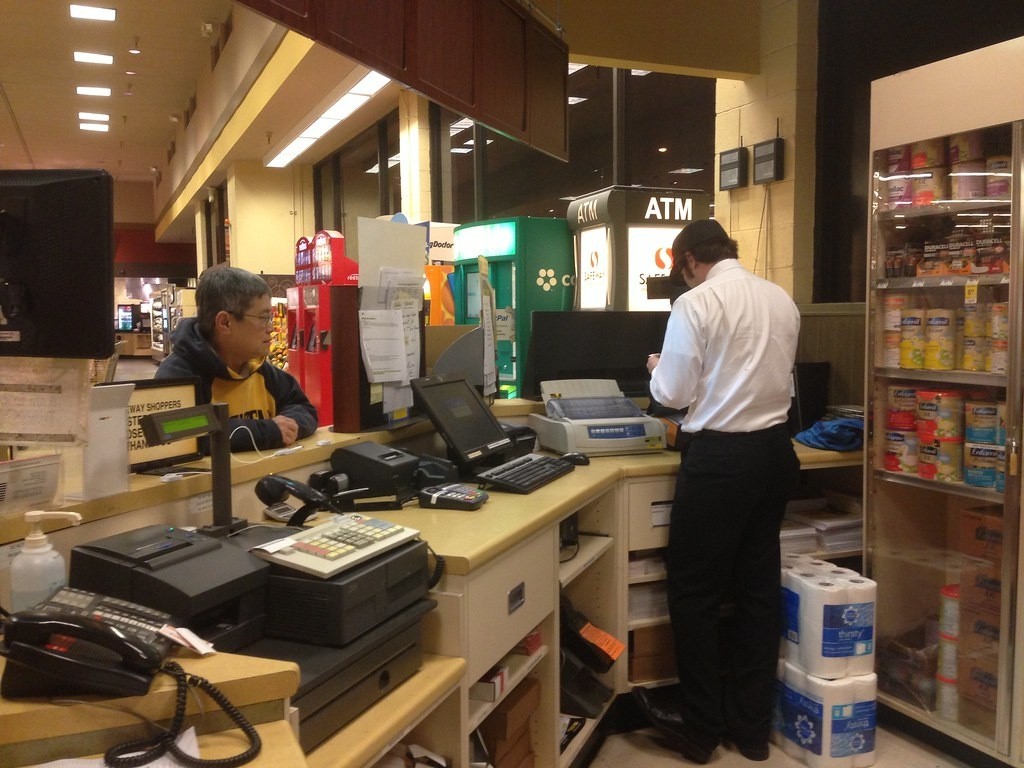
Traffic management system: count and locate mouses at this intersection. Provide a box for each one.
[559,452,590,465]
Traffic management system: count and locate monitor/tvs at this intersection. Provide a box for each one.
[409,371,514,483]
[0,168,114,360]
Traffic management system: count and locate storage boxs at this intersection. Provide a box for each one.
[11,378,137,502]
[480,676,542,740]
[628,658,679,683]
[508,627,542,656]
[490,718,530,766]
[630,623,676,658]
[495,729,531,768]
[517,751,535,768]
[470,662,511,703]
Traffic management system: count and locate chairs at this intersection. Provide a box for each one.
[91,339,128,383]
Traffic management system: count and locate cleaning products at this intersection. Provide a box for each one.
[7,509,85,616]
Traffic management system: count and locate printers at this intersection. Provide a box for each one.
[528,379,666,457]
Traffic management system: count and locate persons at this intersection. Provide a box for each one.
[630,220,801,765]
[154,265,318,456]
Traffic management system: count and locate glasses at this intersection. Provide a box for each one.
[225,309,272,326]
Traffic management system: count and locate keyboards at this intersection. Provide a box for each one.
[251,512,421,580]
[475,453,575,493]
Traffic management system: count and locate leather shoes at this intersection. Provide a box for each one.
[721,714,769,762]
[632,686,713,764]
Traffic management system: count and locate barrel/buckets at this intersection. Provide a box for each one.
[881,293,1008,372]
[878,384,1009,493]
[882,136,1011,201]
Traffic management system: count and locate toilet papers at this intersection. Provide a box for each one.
[769,551,880,768]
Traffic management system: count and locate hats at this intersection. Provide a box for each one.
[668,219,727,284]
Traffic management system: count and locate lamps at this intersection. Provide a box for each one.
[169,111,182,124]
[150,164,159,176]
[200,18,218,39]
[207,185,217,203]
[127,36,142,55]
[122,83,132,95]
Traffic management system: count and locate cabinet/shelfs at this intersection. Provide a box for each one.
[859,35,1024,768]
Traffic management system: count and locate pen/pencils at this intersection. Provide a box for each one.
[287,445,303,451]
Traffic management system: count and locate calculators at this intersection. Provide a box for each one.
[262,502,318,523]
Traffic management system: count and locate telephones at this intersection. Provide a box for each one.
[0,584,181,696]
